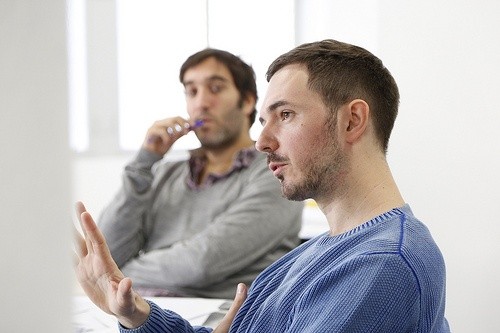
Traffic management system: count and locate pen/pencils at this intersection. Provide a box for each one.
[160,120,204,144]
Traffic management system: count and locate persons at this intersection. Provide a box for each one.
[71,39,451,333]
[96,49,305,300]
